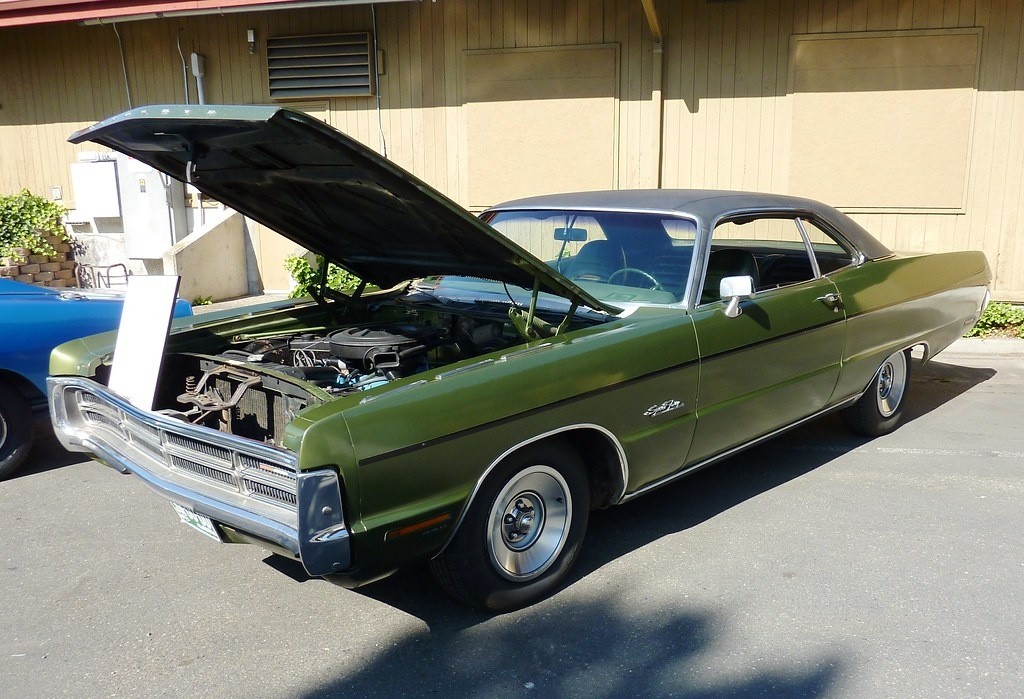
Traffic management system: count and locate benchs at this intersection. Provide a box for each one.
[756,254,835,287]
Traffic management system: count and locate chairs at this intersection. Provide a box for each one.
[563,240,627,286]
[678,248,759,305]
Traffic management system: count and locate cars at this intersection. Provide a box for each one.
[0,278,194,484]
[43,100,990,612]
